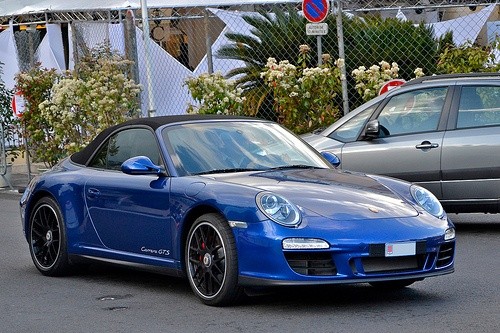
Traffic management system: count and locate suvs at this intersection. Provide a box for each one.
[298,71,499,213]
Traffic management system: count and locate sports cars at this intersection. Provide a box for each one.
[19,116,456,305]
